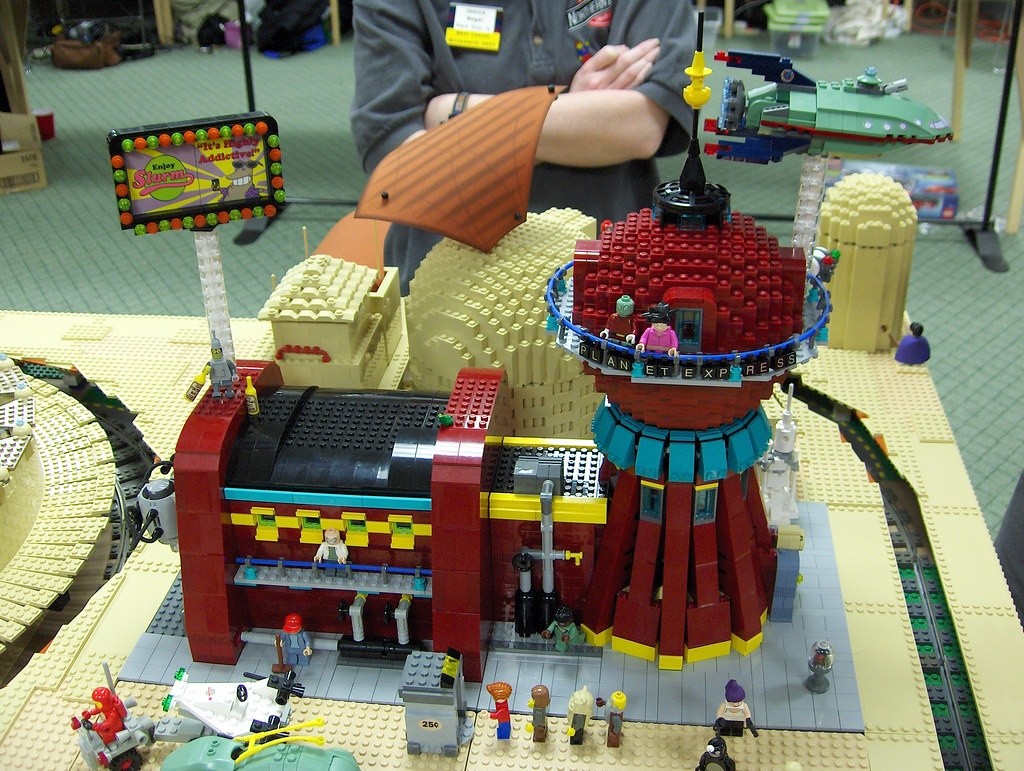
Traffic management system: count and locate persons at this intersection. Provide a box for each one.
[347,0,701,293]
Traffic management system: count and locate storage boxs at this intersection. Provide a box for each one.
[0,113,47,194]
[768,20,822,60]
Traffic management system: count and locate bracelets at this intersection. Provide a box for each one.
[448,90,470,117]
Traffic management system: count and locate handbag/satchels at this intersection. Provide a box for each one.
[198,14,230,46]
[51,32,120,68]
[257,0,329,57]
